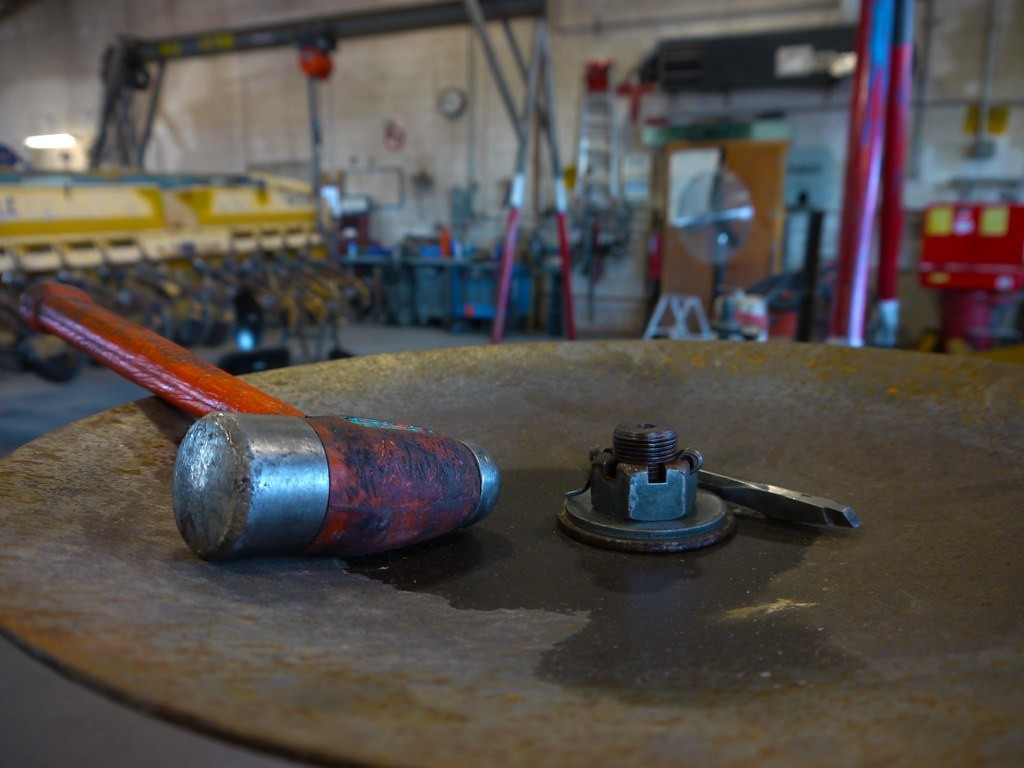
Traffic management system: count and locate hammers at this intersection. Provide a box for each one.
[14,274,502,565]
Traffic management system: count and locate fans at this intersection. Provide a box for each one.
[677,169,752,327]
